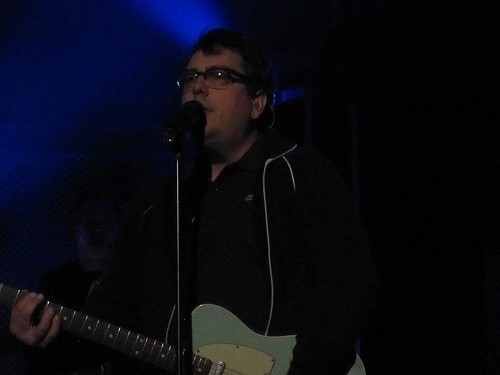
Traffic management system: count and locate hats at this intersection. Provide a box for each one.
[202,28,276,130]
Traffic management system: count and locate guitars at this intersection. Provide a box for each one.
[0,280,375,375]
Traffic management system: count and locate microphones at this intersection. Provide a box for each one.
[161,100,204,144]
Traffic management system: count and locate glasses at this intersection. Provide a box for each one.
[176,67,253,89]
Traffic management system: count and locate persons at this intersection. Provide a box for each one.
[4,27,378,375]
